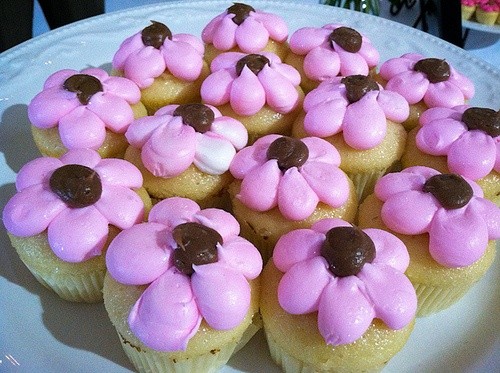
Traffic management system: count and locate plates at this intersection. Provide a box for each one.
[0,0,500,373]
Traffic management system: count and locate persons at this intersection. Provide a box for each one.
[0,0,107,52]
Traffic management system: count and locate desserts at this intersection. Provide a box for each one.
[0,1,499,373]
